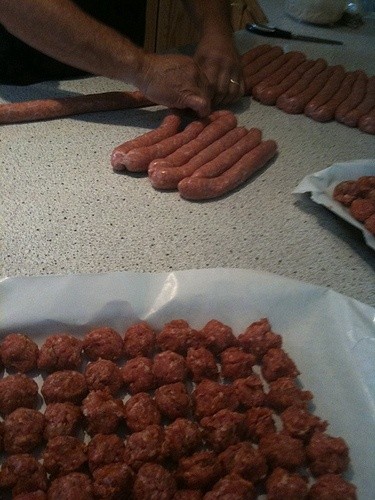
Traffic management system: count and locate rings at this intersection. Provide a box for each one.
[229,78,241,85]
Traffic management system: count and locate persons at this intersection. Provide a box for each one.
[1,0,248,118]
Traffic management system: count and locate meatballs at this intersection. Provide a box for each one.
[0,317,358,500]
[333,176,375,239]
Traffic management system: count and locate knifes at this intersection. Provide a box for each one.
[246,23,344,46]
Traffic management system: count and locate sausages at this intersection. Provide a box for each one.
[0,42,374,199]
